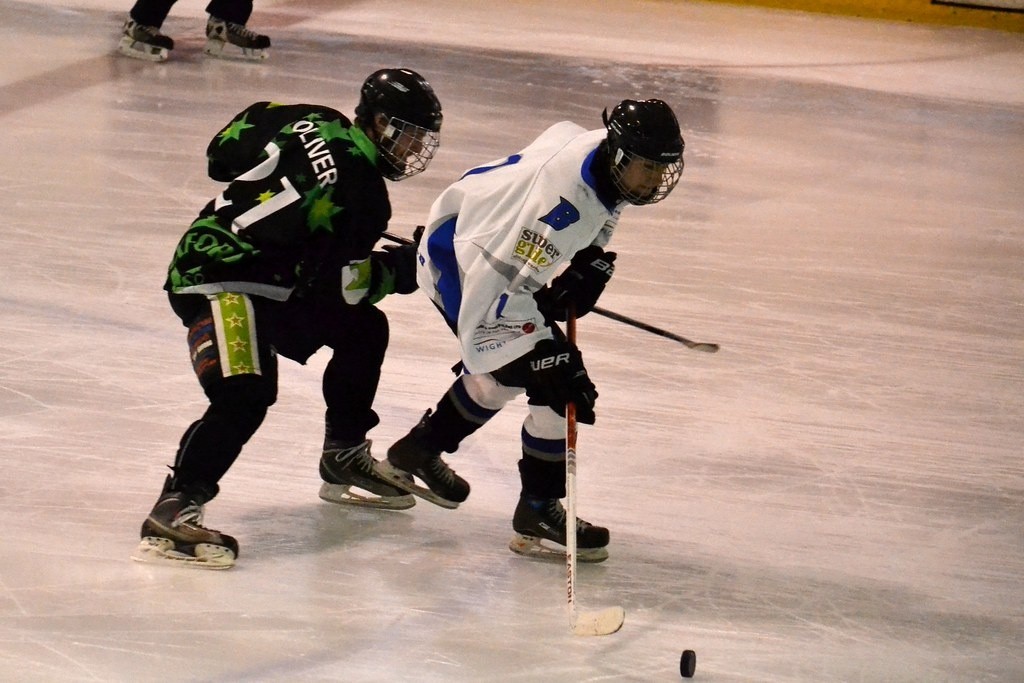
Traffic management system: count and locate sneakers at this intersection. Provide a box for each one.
[115,17,176,62]
[318,410,417,510]
[204,14,271,60]
[372,414,471,510]
[129,473,240,571]
[509,496,610,564]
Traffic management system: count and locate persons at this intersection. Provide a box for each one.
[118,0,271,60]
[373,99,686,561]
[140,69,443,569]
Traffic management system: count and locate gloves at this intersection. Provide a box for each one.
[537,244,616,322]
[392,225,425,295]
[549,366,599,425]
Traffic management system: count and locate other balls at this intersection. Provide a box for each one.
[680,650,698,678]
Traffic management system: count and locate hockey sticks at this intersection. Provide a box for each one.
[382,229,723,354]
[566,304,626,637]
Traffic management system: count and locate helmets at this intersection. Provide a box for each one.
[353,67,444,183]
[606,99,686,207]
